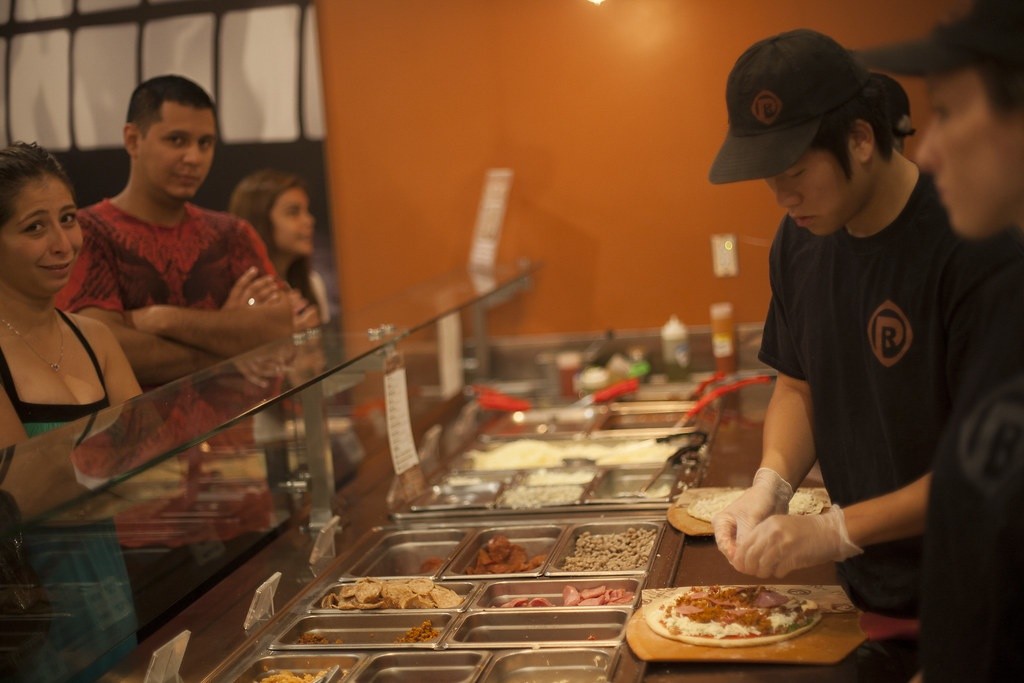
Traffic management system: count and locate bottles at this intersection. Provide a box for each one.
[661,314,689,378]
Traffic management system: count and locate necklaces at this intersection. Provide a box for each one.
[0,301,65,372]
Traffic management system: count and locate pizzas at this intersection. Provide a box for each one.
[690,491,823,522]
[646,588,822,646]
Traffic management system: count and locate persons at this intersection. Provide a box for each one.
[53,73,294,683]
[225,169,332,559]
[711,28,959,682]
[850,0,1024,682]
[0,137,142,683]
[860,72,919,159]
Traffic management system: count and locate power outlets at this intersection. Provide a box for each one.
[708,234,738,277]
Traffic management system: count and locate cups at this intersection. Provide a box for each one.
[710,303,735,373]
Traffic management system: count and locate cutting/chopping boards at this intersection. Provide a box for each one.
[667,488,830,535]
[624,601,922,666]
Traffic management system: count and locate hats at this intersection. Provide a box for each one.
[710,29,869,184]
[859,0,1024,108]
[868,72,917,136]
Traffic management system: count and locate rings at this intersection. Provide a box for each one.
[247,297,257,307]
